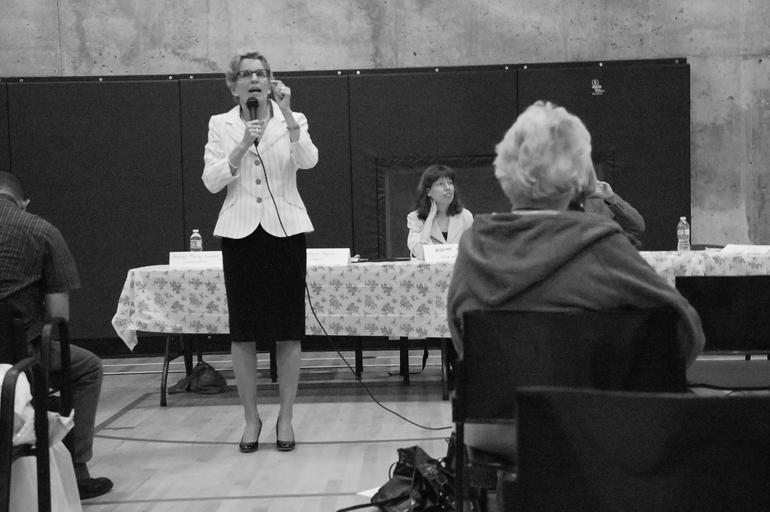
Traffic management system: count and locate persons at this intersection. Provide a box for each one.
[407,162,474,260]
[450,97,701,480]
[205,50,319,453]
[573,172,646,248]
[0,166,114,500]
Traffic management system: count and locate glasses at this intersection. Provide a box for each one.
[229,66,272,81]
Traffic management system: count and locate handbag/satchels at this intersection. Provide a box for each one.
[369,442,475,512]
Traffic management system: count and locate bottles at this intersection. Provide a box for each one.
[189,229,202,251]
[676,216,690,250]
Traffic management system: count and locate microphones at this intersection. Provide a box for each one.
[246,96,261,147]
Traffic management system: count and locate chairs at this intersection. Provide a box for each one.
[167,249,225,385]
[497,387,770,512]
[0,357,51,512]
[269,246,365,382]
[450,310,683,511]
[0,304,72,417]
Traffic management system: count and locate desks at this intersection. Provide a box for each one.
[110,249,769,406]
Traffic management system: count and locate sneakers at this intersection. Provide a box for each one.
[77,477,114,500]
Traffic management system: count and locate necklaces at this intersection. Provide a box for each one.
[240,109,271,124]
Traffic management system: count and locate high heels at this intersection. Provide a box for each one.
[273,415,298,451]
[238,419,263,454]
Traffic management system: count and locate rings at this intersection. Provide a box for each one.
[256,129,259,134]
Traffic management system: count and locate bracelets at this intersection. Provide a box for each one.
[286,126,300,132]
[228,158,240,170]
